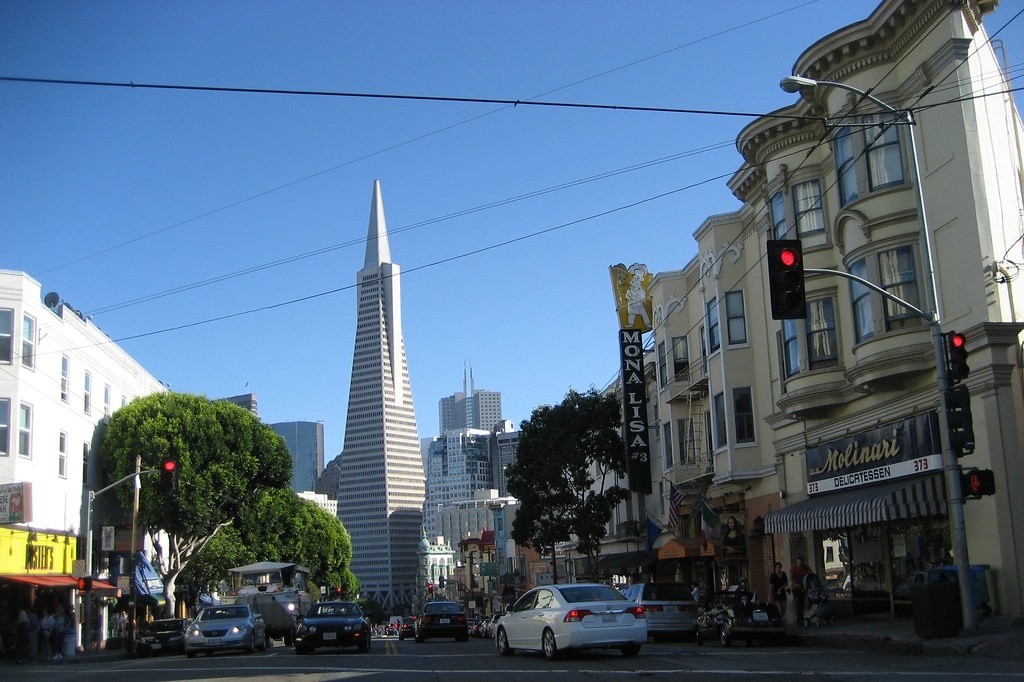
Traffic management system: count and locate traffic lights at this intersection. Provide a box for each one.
[966,468,996,496]
[945,382,975,457]
[948,331,970,379]
[766,238,809,320]
[160,456,179,496]
[428,583,433,593]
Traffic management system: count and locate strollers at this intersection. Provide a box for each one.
[801,573,835,627]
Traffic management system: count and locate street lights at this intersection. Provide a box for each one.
[778,74,976,636]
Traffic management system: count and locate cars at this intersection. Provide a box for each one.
[398,616,417,640]
[467,614,506,639]
[295,600,371,654]
[414,601,468,643]
[494,582,648,661]
[386,622,397,636]
[695,589,785,649]
[184,603,265,658]
[136,617,193,659]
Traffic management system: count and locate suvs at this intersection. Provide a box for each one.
[619,582,697,642]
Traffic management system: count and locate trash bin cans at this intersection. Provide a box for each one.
[911,570,962,639]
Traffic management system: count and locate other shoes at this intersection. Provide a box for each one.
[52,653,63,660]
[40,655,52,662]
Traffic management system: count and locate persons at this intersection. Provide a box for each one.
[41,606,72,662]
[15,610,32,664]
[118,611,128,636]
[690,582,700,602]
[768,562,790,618]
[789,556,813,627]
[806,580,827,601]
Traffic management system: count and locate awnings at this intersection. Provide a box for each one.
[130,586,167,608]
[658,538,723,560]
[764,469,948,534]
[5,574,121,597]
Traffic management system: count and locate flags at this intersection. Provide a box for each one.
[645,515,663,556]
[700,502,718,553]
[668,482,687,528]
